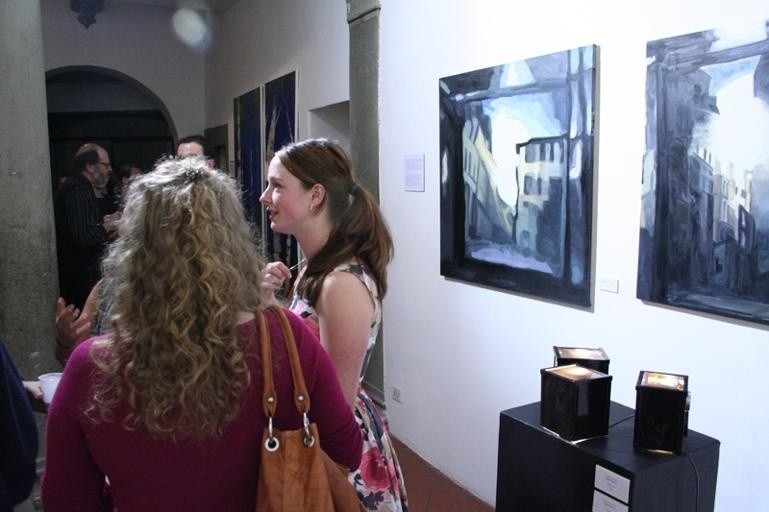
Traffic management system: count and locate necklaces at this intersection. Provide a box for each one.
[287,257,312,271]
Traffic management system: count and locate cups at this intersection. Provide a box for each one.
[38,371,64,414]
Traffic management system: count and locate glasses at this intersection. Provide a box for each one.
[95,162,110,168]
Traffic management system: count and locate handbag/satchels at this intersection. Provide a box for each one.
[255,304,369,512]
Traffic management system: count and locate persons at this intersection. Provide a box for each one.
[0,339,58,512]
[255,138,412,512]
[38,155,366,512]
